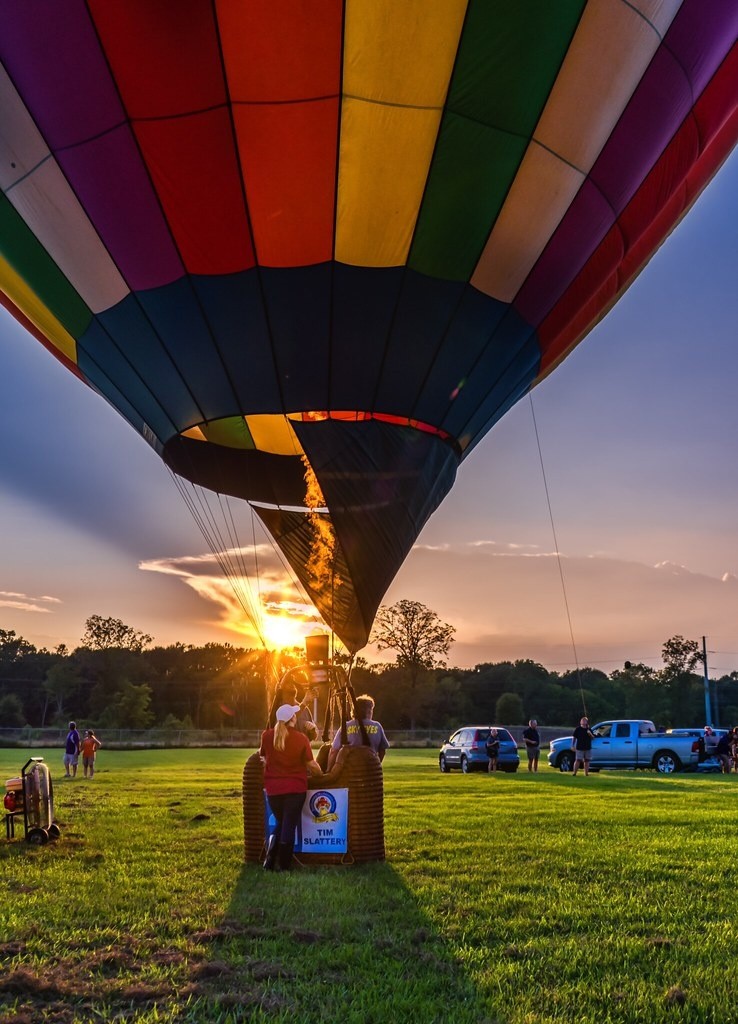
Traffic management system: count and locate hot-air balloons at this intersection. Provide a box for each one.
[4,1,736,862]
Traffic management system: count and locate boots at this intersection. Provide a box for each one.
[276,848,304,872]
[263,835,278,868]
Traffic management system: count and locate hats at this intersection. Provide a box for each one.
[276,703,300,724]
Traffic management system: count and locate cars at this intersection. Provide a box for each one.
[438,727,519,774]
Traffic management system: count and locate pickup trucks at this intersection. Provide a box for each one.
[548,720,704,773]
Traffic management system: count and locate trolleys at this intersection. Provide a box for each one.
[3,756,60,845]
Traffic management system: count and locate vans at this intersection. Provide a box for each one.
[667,728,729,754]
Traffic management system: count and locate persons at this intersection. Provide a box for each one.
[571,717,594,777]
[269,674,319,744]
[63,722,80,778]
[324,694,389,774]
[78,729,101,781]
[703,724,738,773]
[486,729,500,773]
[522,720,540,773]
[257,705,322,872]
